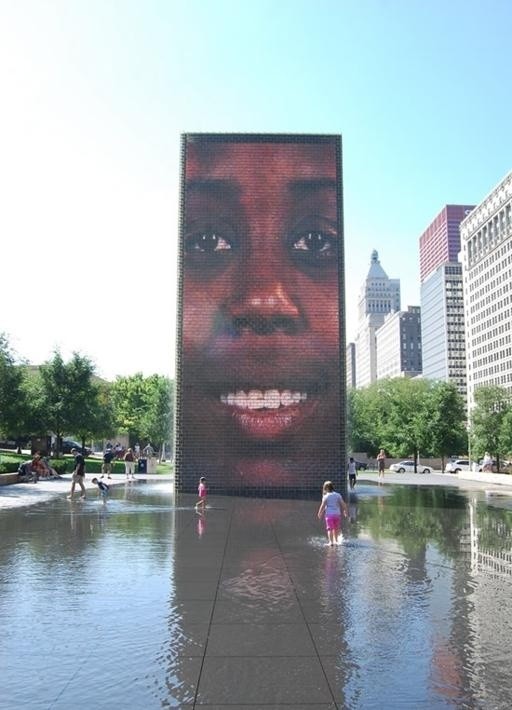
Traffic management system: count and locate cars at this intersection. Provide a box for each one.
[348,458,369,471]
[51,441,92,457]
[390,461,433,474]
[446,460,512,473]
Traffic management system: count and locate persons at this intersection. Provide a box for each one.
[122,447,137,480]
[105,440,155,460]
[100,449,113,479]
[193,476,208,510]
[376,449,387,479]
[91,477,109,505]
[194,511,209,541]
[17,448,63,482]
[346,457,358,490]
[66,447,87,501]
[317,481,348,547]
[177,136,342,502]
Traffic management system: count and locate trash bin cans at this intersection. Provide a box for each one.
[138,459,147,474]
[147,457,157,473]
